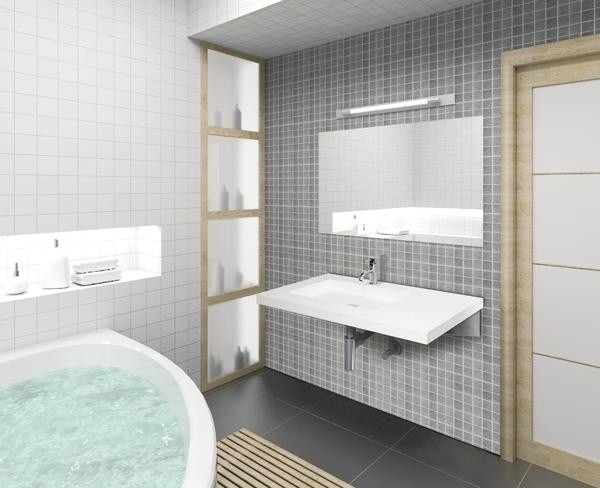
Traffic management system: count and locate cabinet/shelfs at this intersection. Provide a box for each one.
[199,40,268,392]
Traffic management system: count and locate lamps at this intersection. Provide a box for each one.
[335,93,456,121]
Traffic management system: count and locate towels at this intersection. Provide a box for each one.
[71,257,121,286]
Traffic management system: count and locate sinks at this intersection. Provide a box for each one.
[255,272,484,345]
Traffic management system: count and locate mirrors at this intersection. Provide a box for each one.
[317,114,485,249]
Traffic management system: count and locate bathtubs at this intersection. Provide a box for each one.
[0,327,218,488]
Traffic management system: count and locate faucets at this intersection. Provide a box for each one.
[359,257,377,284]
